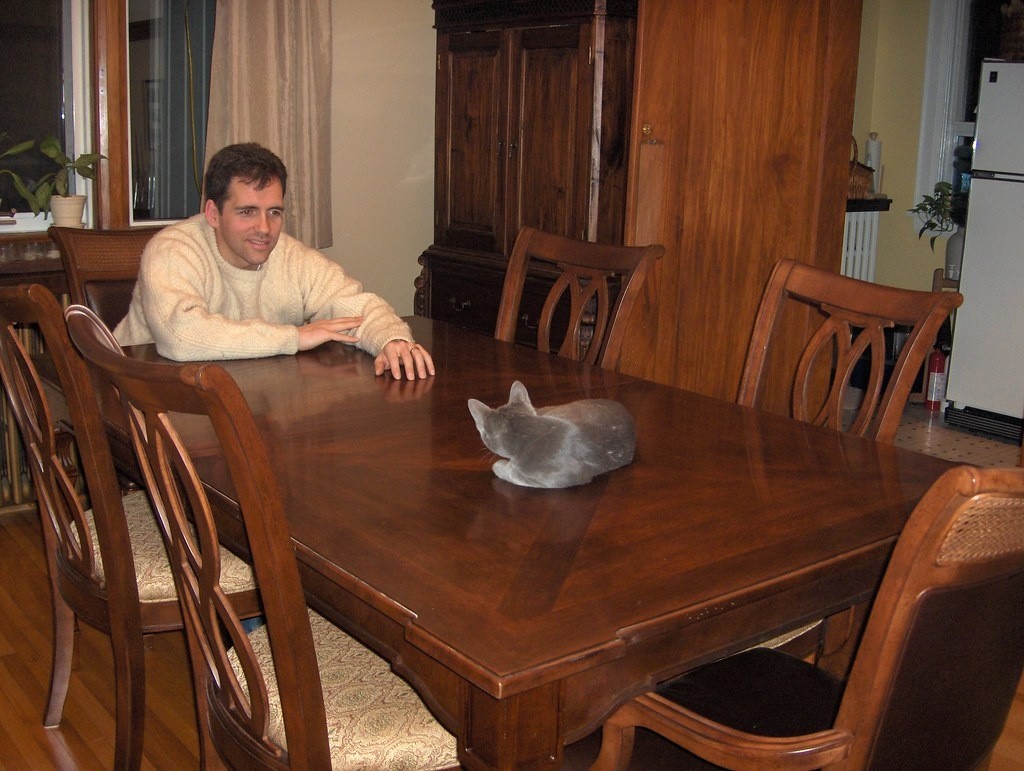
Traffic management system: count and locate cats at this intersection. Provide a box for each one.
[467,380,635,487]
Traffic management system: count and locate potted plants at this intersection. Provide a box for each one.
[909,180,966,280]
[0,134,109,228]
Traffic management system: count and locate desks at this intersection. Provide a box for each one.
[31,316,968,771]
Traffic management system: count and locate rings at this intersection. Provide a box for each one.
[410,348,420,353]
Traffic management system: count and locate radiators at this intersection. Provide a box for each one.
[840,211,880,283]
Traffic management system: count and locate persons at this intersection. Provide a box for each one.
[110,143,436,635]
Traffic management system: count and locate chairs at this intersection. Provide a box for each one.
[602,466,1024,771]
[0,281,263,771]
[738,260,964,646]
[59,303,460,771]
[492,227,666,367]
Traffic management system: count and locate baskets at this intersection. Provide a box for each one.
[846,135,874,199]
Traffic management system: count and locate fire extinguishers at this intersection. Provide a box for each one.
[924,344,946,411]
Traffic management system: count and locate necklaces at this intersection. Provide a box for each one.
[256,264,261,270]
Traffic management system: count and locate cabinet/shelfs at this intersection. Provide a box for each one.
[0,229,106,516]
[425,0,863,423]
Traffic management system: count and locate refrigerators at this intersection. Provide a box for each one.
[945,57,1024,443]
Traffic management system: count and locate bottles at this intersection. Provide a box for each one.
[864,132,882,195]
